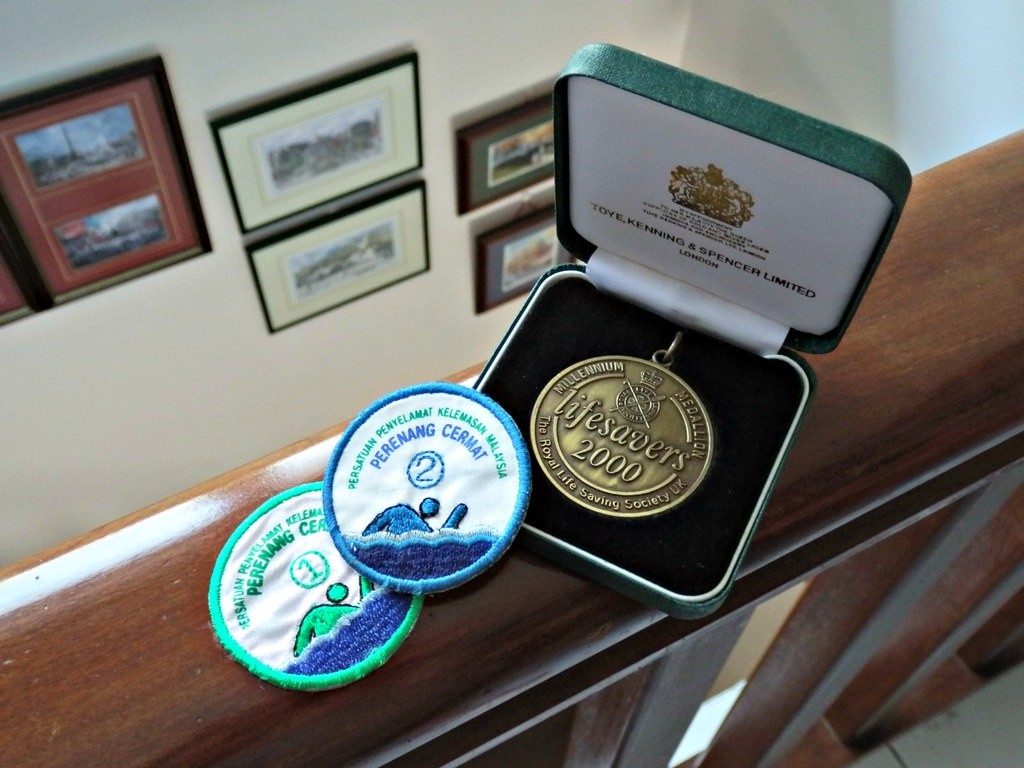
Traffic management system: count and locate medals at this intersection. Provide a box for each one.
[529,331,713,517]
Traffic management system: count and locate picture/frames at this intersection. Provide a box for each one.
[245,179,430,333]
[456,92,555,216]
[0,54,214,328]
[209,52,424,235]
[474,201,577,315]
